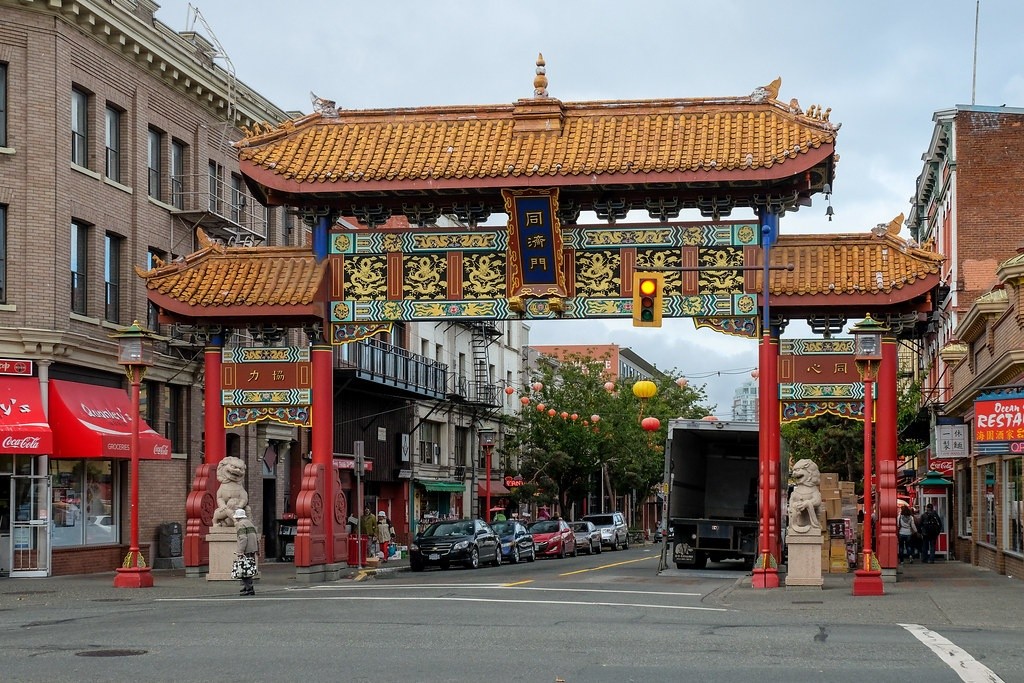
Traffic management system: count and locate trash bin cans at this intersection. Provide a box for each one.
[347,534,368,567]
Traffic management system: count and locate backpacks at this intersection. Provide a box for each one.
[923,512,938,536]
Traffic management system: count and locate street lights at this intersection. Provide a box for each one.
[475,421,498,522]
[846,313,892,597]
[107,320,172,588]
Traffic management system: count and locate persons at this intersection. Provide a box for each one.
[231,509,258,596]
[539,508,550,520]
[551,512,562,521]
[896,504,943,564]
[356,506,395,563]
[857,510,864,523]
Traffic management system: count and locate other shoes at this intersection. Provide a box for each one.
[910,555,913,564]
[930,561,934,564]
[239,586,246,592]
[240,589,256,597]
[901,562,904,565]
[382,560,387,563]
[921,560,928,563]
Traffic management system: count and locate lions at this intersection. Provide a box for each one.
[789,459,820,524]
[214,456,248,525]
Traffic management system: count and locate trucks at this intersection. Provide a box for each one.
[656,418,790,570]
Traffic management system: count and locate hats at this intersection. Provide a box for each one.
[233,509,247,518]
[539,505,549,508]
[378,511,386,517]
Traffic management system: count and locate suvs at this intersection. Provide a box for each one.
[578,511,630,551]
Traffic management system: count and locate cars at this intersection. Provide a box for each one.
[566,521,602,555]
[525,519,578,559]
[486,520,536,564]
[408,518,503,572]
[654,521,674,542]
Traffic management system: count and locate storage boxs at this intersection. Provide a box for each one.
[817,474,864,573]
[389,546,407,561]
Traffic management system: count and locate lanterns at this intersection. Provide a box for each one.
[605,382,614,392]
[537,404,545,412]
[675,378,687,387]
[752,370,760,378]
[533,382,544,391]
[521,397,529,404]
[548,408,556,417]
[641,417,661,433]
[505,387,514,394]
[632,381,657,403]
[561,412,600,432]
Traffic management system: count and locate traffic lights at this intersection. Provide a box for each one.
[633,272,663,327]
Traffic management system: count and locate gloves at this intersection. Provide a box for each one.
[391,533,395,537]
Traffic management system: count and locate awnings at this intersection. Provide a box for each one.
[478,479,511,497]
[0,376,172,461]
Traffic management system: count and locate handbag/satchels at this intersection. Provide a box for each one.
[897,514,901,535]
[231,554,258,579]
[387,538,396,557]
